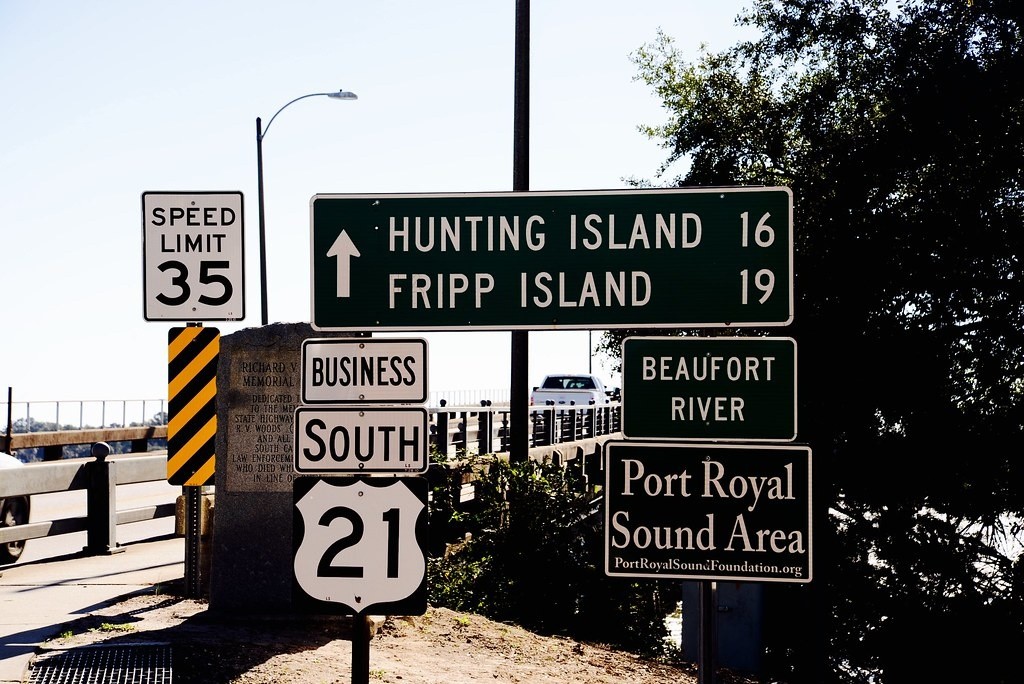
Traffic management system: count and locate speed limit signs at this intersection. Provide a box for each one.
[139,188,247,331]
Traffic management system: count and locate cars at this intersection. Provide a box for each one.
[530,374,615,416]
[0,436,34,563]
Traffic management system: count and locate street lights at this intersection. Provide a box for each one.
[253,88,359,328]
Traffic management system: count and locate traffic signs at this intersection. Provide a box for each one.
[307,183,802,330]
